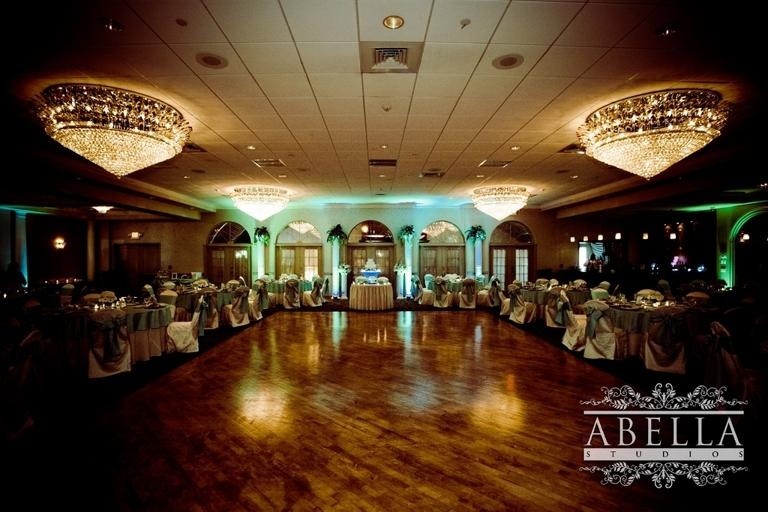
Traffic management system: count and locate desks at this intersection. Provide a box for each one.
[349,281,395,310]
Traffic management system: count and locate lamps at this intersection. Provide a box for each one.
[31,83,195,180]
[574,89,730,182]
[214,182,300,223]
[461,182,535,224]
